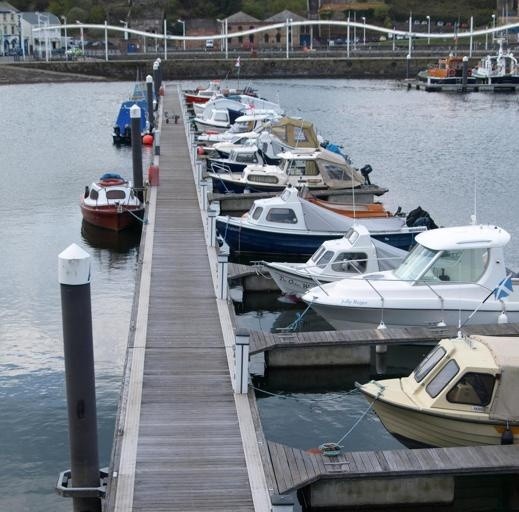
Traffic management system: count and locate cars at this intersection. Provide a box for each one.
[81,40,113,48]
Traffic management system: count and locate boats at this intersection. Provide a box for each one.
[79,173,144,232]
[415,37,519,91]
[113,83,157,143]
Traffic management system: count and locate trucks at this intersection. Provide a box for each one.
[62,37,80,48]
[206,40,213,48]
